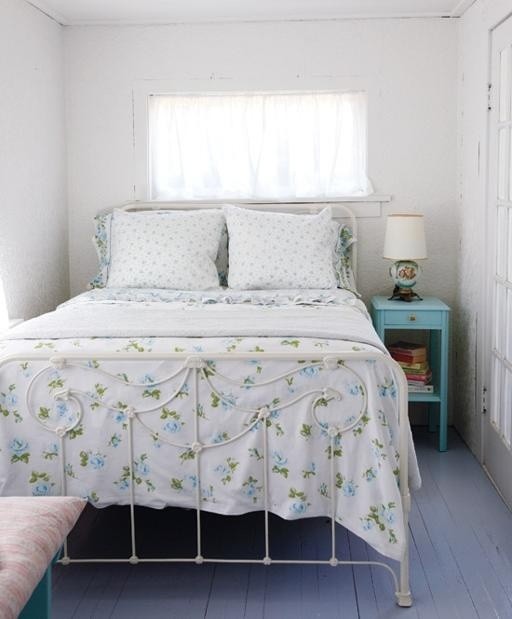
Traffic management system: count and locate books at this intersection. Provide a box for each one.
[387,341,434,393]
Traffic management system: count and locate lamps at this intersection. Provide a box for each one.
[381,212,426,301]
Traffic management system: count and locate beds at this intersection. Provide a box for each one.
[1,200,423,608]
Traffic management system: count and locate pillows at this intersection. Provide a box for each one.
[223,202,339,291]
[107,209,226,290]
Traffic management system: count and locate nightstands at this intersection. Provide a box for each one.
[373,295,452,453]
